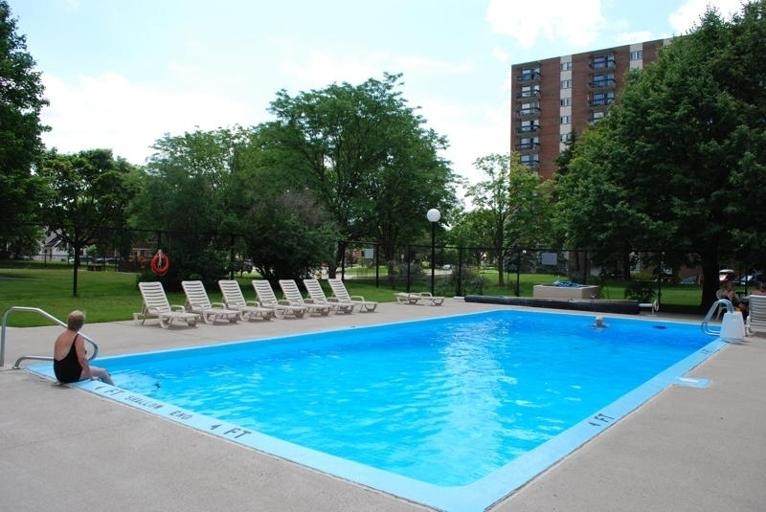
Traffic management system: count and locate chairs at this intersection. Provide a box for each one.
[394,291,444,306]
[715,289,741,320]
[133,278,379,329]
[746,295,766,337]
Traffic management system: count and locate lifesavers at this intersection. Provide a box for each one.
[151,255,169,277]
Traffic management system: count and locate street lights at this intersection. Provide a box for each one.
[426,208,441,296]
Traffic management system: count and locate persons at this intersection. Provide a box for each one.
[720,281,766,324]
[591,317,607,328]
[52,309,116,386]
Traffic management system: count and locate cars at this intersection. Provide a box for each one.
[719,269,766,290]
